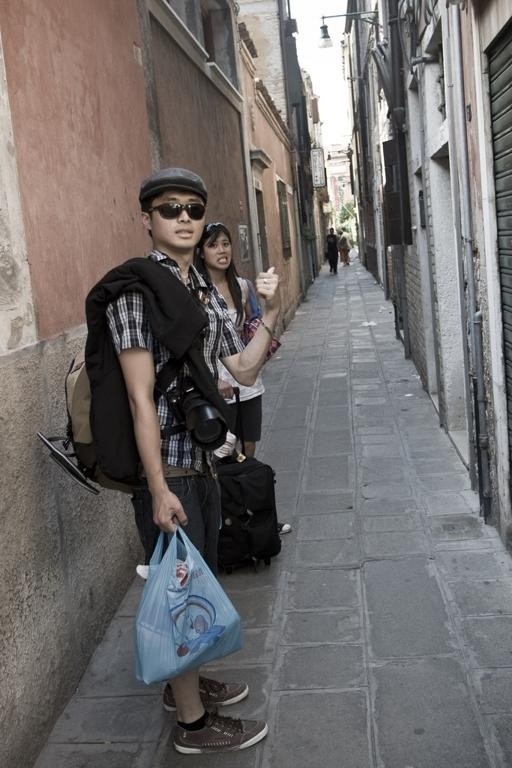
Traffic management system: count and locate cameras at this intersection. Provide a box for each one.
[167,376,227,451]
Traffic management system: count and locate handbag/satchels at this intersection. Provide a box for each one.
[242,317,283,362]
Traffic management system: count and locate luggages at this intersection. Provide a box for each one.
[213,385,282,573]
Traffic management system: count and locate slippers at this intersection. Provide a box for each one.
[37,433,102,498]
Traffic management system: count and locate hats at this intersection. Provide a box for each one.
[138,166,209,204]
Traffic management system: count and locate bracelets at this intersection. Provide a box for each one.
[259,318,273,340]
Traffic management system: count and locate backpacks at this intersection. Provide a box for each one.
[62,347,185,498]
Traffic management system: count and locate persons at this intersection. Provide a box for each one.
[193,220,293,535]
[325,226,340,275]
[84,165,278,757]
[336,231,350,267]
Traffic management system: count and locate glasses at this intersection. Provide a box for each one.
[145,200,206,221]
[205,222,225,234]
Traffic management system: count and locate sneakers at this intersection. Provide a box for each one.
[277,522,292,534]
[166,714,268,757]
[161,672,252,715]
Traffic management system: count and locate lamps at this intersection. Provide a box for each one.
[318,10,380,52]
